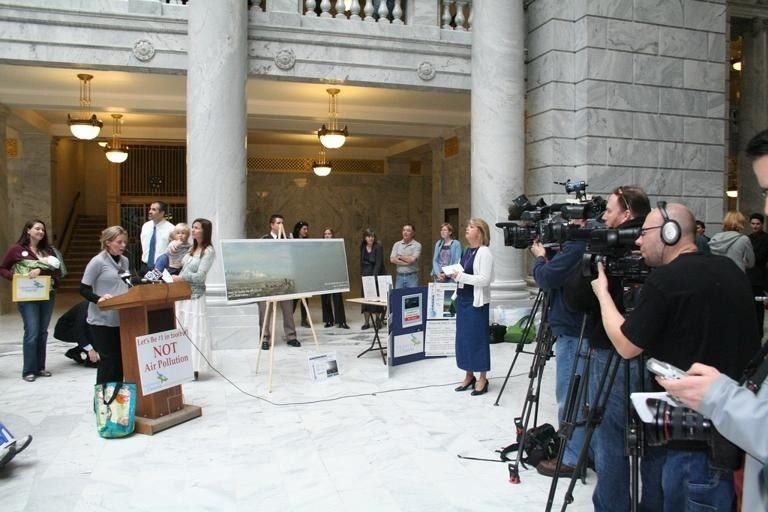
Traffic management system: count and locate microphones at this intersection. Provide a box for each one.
[132,277,161,283]
[118,269,135,287]
[140,269,157,280]
[150,266,167,283]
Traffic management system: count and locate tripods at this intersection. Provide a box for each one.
[494,287,544,406]
[560,300,644,512]
[509,299,556,484]
[545,311,587,512]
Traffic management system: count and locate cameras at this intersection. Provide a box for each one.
[644,397,743,471]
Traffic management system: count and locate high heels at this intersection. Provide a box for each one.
[470,378,489,396]
[455,376,476,391]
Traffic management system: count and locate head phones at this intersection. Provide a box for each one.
[657,201,681,246]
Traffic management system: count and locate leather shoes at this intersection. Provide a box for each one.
[300,319,312,329]
[338,321,351,330]
[23,374,35,382]
[323,320,335,328]
[37,370,51,376]
[287,339,301,348]
[261,341,270,351]
[361,321,381,330]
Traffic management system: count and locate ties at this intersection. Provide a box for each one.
[147,225,156,269]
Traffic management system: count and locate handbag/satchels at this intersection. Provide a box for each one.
[94,383,137,439]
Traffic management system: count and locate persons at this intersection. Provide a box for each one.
[154,222,191,276]
[0,218,60,382]
[79,226,131,412]
[531,127,768,512]
[0,421,32,470]
[449,217,493,396]
[53,301,100,368]
[175,217,214,380]
[138,201,176,277]
[260,214,461,351]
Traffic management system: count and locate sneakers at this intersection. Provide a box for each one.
[0,446,15,467]
[534,456,583,481]
[64,349,86,364]
[15,433,32,452]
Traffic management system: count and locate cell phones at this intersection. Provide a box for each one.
[647,358,694,381]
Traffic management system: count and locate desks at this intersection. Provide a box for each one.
[346,297,388,365]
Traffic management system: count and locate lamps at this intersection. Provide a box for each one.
[67,74,103,141]
[312,146,333,177]
[318,88,348,150]
[731,57,742,72]
[105,114,129,165]
[726,185,738,198]
